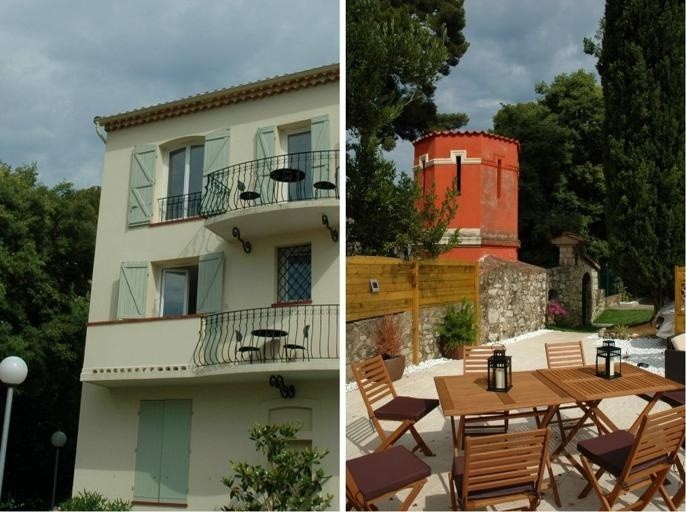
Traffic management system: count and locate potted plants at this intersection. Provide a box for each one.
[433,298,483,360]
[366,309,405,381]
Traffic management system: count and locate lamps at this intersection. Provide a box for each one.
[232,227,251,253]
[269,375,295,399]
[322,213,338,242]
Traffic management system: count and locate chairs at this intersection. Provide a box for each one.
[235,180,262,209]
[314,167,339,198]
[235,325,310,363]
[346,341,684,511]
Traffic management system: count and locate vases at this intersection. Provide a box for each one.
[546,311,557,326]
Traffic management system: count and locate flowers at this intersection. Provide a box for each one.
[548,299,567,317]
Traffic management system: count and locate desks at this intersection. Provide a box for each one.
[270,168,305,203]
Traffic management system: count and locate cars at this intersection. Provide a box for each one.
[653,297,684,339]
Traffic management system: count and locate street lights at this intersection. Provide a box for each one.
[0,356,28,497]
[51,431,67,508]
